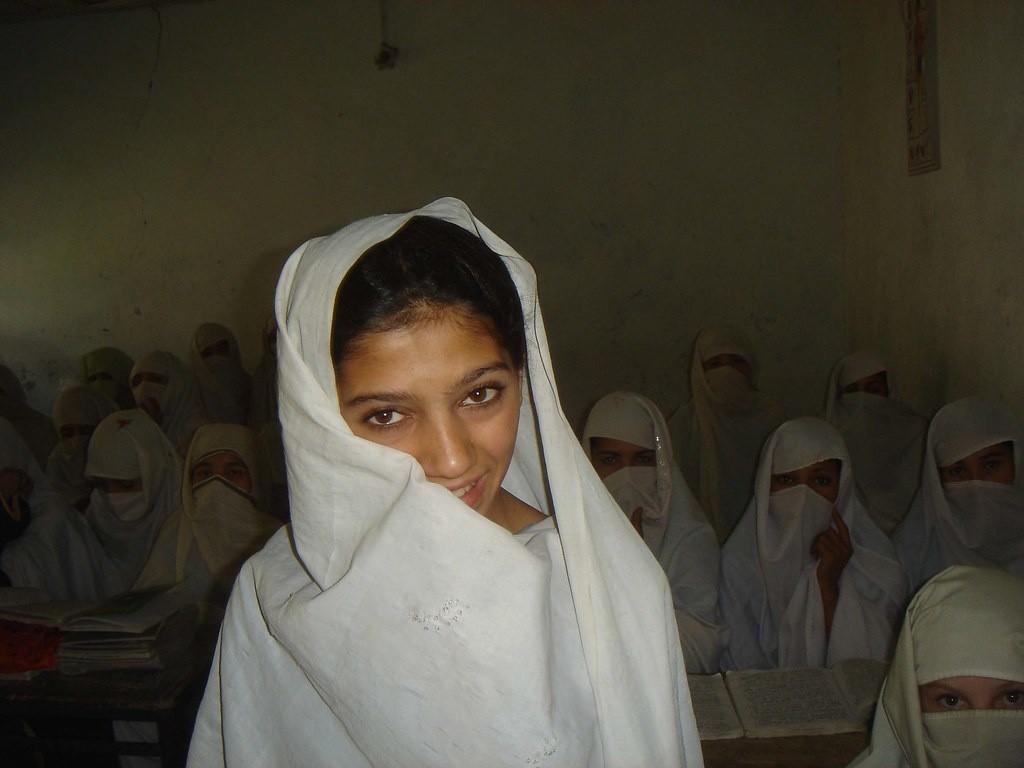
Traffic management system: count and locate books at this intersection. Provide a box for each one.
[0,587,197,711]
[690,656,893,741]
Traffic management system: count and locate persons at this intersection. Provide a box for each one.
[181,198,700,768]
[81,347,136,411]
[127,351,206,460]
[585,390,730,675]
[815,351,929,537]
[887,398,1024,599]
[83,409,181,768]
[0,365,58,474]
[132,423,286,631]
[666,324,788,546]
[0,417,108,601]
[717,416,909,674]
[180,324,252,425]
[848,565,1024,768]
[45,388,100,515]
[248,310,286,498]
